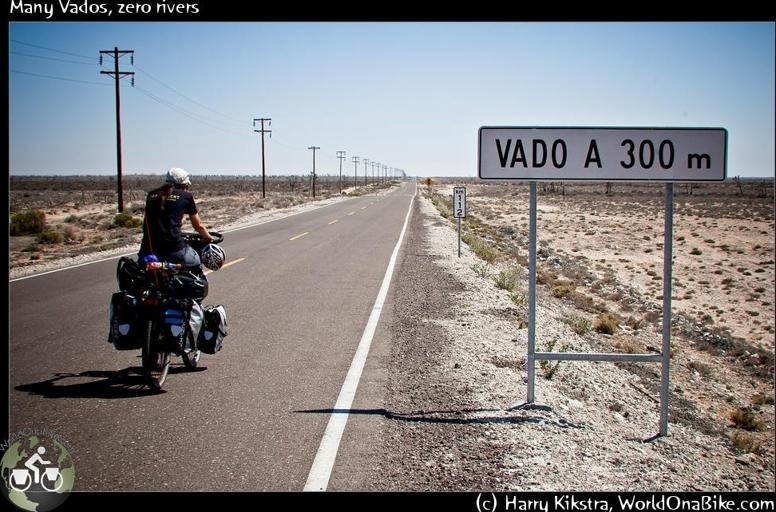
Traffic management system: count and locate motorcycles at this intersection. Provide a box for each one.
[106,230,229,396]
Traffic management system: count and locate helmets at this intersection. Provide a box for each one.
[201,243,226,271]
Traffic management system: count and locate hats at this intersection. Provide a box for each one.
[166,167,192,187]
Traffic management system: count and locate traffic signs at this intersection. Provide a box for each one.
[479,128,727,183]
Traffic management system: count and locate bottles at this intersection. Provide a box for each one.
[146,263,182,271]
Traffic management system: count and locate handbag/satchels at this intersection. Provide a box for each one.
[106,255,227,354]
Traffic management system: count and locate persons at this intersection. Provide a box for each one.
[138,168,214,268]
